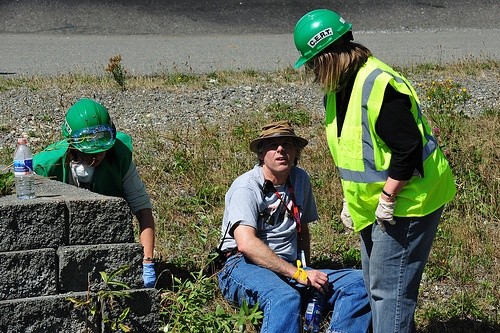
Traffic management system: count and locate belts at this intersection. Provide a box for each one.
[224,249,240,261]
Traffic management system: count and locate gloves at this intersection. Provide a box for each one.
[375,197,396,233]
[142,263,156,288]
[340,197,354,231]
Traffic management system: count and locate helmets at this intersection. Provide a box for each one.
[293,8,353,69]
[61,97,117,154]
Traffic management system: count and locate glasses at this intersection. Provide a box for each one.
[72,124,114,148]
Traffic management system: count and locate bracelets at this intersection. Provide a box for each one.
[292,260,306,281]
[142,257,156,261]
[382,189,397,198]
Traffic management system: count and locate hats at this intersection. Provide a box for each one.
[250,120,308,154]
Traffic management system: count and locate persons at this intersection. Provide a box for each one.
[293,9,458,333]
[32,98,157,288]
[216,119,373,333]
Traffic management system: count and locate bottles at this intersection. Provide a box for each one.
[304,292,322,333]
[14,138,35,200]
